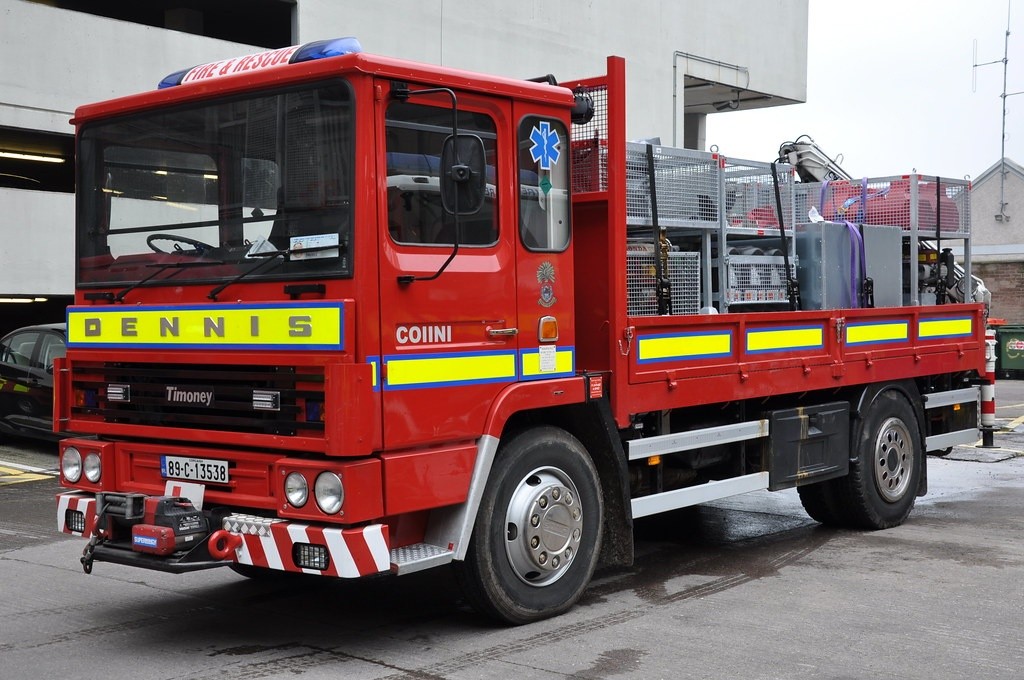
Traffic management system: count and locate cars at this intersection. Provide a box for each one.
[0,321,92,443]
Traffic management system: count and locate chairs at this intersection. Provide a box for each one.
[48,343,66,366]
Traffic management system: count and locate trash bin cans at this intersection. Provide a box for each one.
[985,317,1024,380]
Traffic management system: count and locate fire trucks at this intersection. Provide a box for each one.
[50,35,1000,622]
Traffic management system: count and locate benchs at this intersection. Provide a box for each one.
[2,341,36,366]
